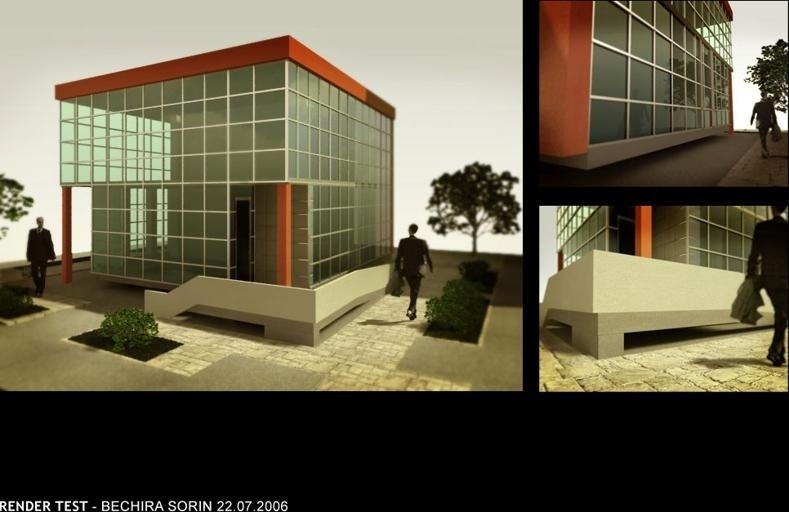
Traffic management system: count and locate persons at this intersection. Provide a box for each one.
[24,216,58,300]
[744,204,788,367]
[392,223,435,320]
[749,89,780,159]
